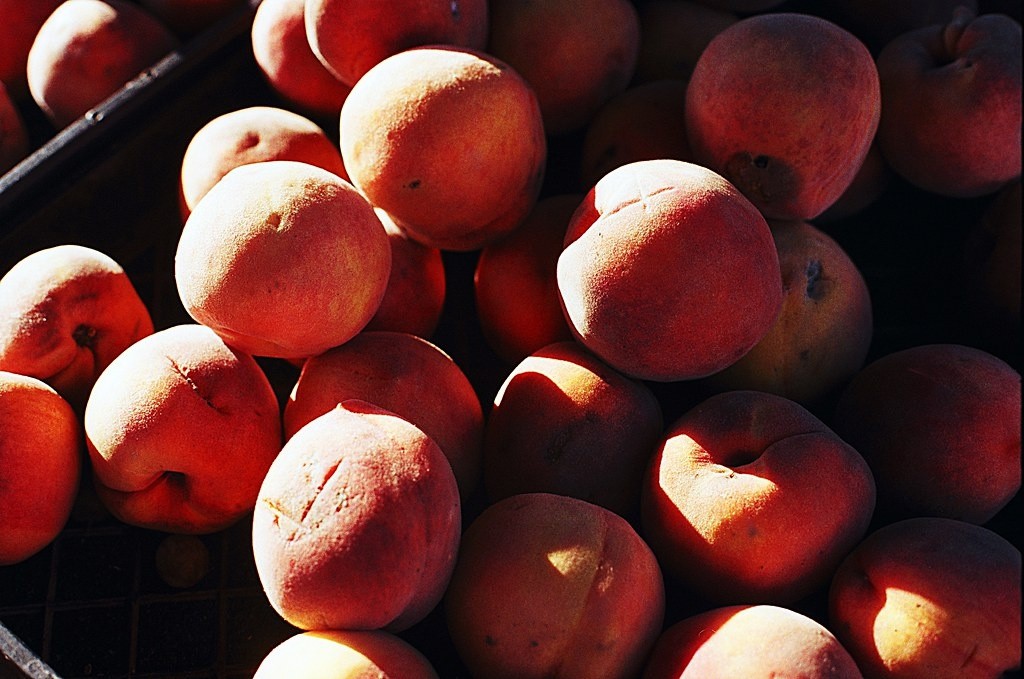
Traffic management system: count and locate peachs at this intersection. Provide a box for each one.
[0,0,1024,679]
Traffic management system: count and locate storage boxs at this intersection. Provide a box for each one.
[0,0,264,679]
